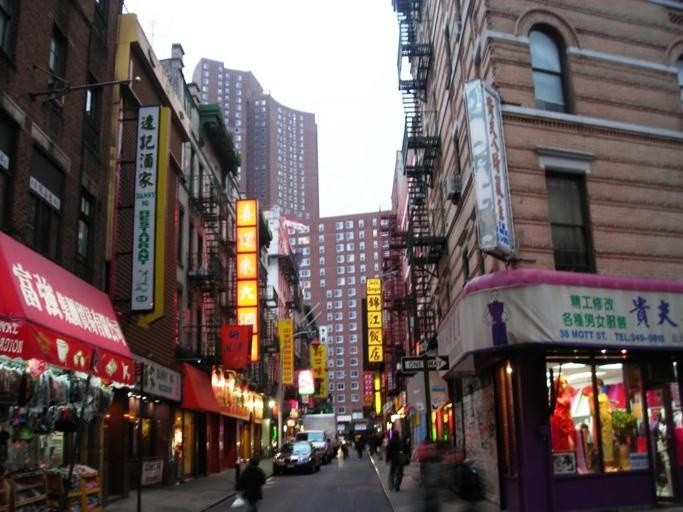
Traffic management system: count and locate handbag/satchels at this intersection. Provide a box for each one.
[230,492,246,510]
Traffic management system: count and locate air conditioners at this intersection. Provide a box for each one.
[442,175,456,200]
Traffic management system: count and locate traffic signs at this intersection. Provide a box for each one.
[401,354,449,373]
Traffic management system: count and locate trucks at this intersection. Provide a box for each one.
[302,413,341,458]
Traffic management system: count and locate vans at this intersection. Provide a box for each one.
[294,429,332,465]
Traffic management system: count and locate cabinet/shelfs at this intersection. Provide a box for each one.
[1,465,104,512]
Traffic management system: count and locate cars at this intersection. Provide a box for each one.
[272,439,321,475]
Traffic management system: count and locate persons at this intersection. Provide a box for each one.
[355,436,363,460]
[340,438,349,461]
[367,430,378,455]
[552,376,578,451]
[385,429,409,492]
[589,378,615,465]
[232,456,267,512]
